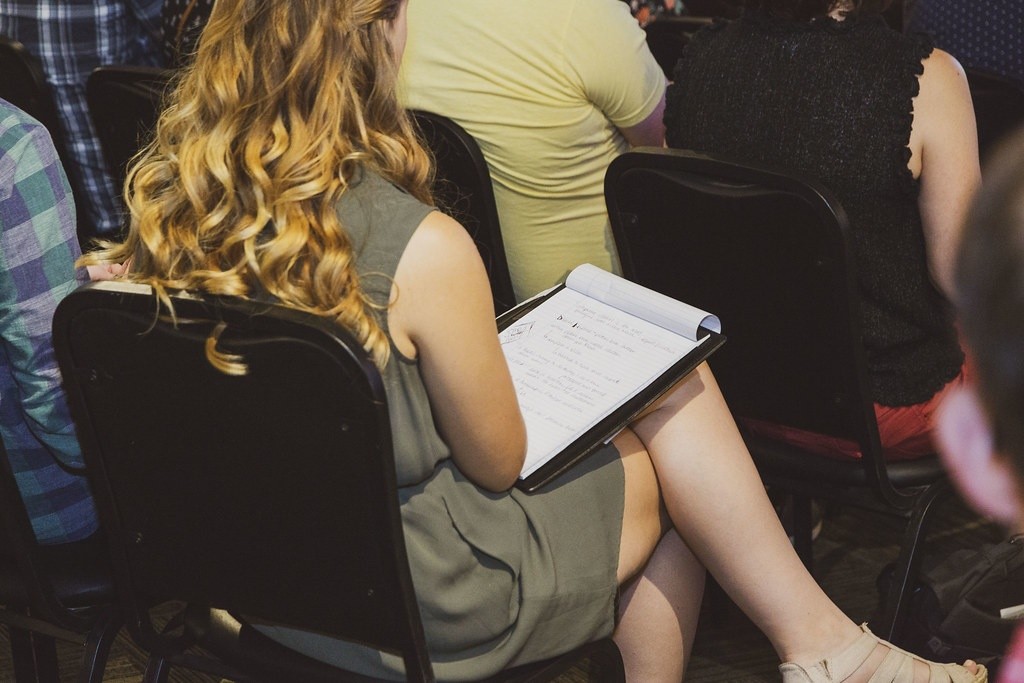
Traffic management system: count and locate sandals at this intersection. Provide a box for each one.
[778,622,988,683]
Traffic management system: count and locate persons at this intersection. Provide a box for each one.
[1,96,129,682]
[74,0,990,683]
[0,1,173,241]
[933,124,1023,683]
[661,1,983,465]
[398,1,674,308]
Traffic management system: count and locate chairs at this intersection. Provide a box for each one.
[0,14,1024,683]
